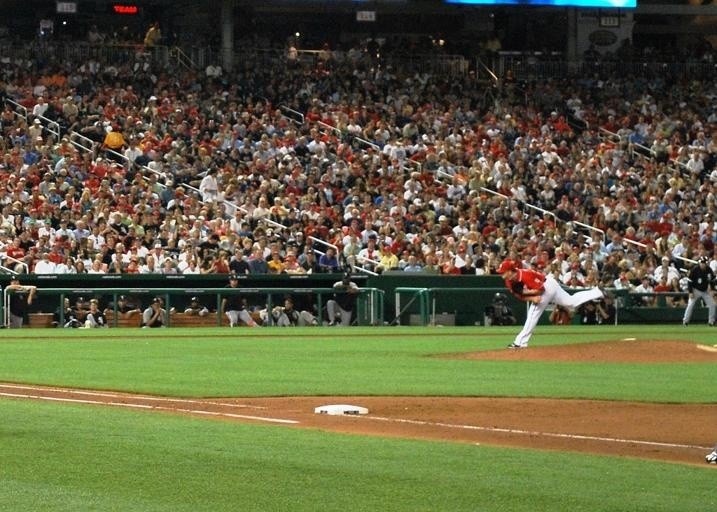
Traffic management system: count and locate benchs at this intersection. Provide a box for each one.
[28,309,263,326]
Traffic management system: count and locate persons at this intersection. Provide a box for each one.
[704,445,717,464]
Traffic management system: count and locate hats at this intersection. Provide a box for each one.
[496,260,516,273]
[227,271,238,281]
[152,296,201,304]
[344,271,350,279]
[77,297,86,304]
[699,255,709,264]
[117,295,129,302]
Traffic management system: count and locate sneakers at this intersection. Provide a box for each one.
[598,284,615,298]
[506,341,528,348]
